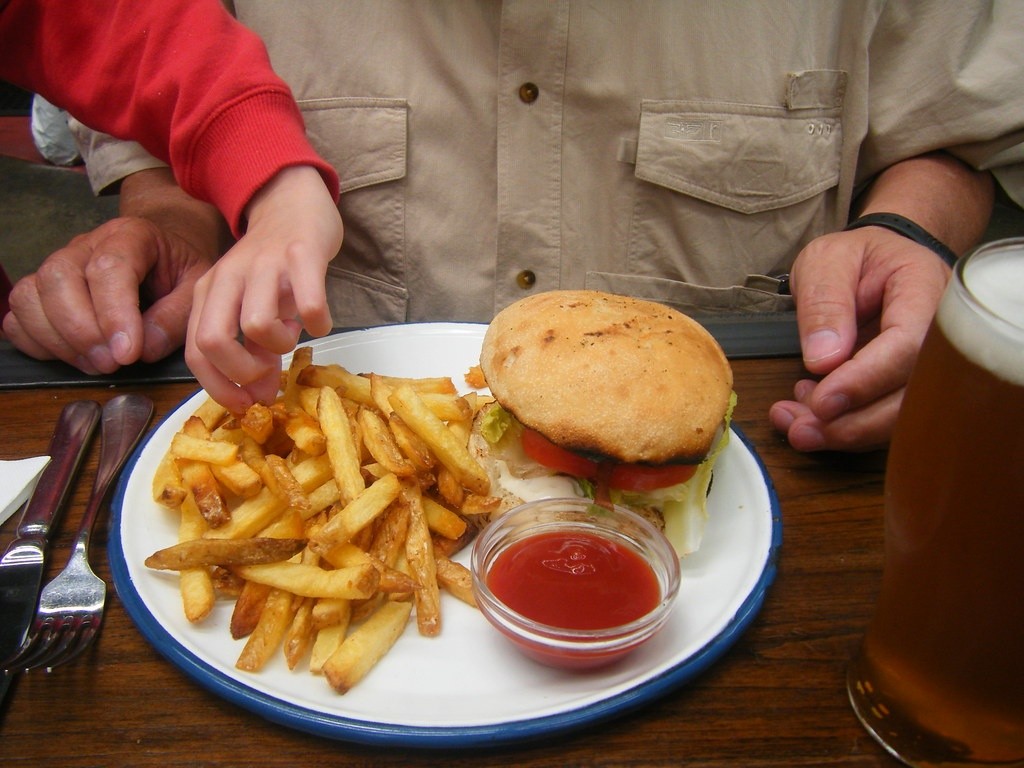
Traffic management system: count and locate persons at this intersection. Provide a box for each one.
[0,0,1024,453]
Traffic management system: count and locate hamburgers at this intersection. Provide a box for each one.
[463,288,738,560]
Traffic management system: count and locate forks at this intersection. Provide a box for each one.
[1,394,153,674]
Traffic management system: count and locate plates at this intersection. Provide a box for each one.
[110,323,782,746]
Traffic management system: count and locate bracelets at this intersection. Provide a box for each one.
[843,212,958,270]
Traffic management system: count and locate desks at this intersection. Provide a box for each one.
[0,302,897,768]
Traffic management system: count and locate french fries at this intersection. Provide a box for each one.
[140,346,503,695]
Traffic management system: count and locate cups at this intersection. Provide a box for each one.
[845,234,1023,768]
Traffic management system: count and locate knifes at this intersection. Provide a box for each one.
[0,398,101,713]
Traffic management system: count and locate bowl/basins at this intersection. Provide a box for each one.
[470,497,682,672]
